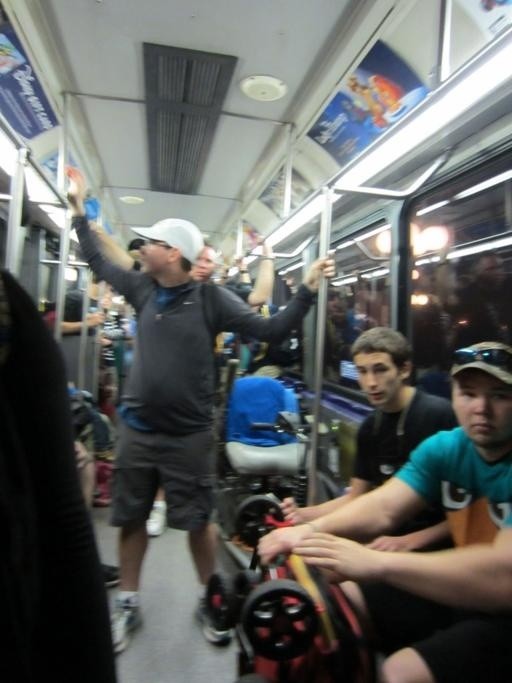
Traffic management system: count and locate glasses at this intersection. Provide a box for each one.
[455,349,510,368]
[144,240,171,249]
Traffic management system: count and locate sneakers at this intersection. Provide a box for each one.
[146,506,167,536]
[99,564,120,587]
[197,602,232,644]
[110,604,139,653]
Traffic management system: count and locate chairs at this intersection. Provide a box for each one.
[225,372,308,478]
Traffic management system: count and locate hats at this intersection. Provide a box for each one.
[132,219,205,264]
[451,341,511,383]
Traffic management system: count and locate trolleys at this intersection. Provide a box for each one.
[202,495,378,681]
